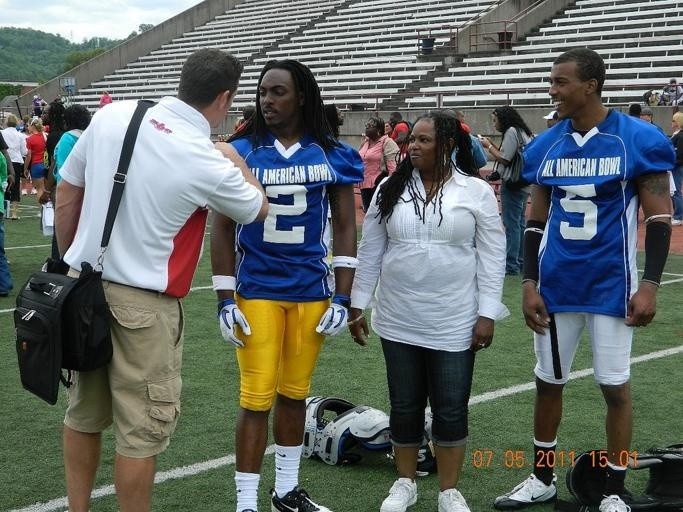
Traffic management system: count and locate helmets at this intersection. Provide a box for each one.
[640,107,654,115]
[301,396,393,466]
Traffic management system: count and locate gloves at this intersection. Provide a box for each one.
[316,294,352,338]
[216,297,252,348]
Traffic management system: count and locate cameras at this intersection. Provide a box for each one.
[486,172,499,181]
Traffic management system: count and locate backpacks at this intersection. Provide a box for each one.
[406,120,415,131]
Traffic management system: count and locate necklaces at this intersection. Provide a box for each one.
[424,167,451,193]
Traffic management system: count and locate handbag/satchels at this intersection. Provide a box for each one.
[380,155,402,172]
[502,145,532,191]
[14,261,113,406]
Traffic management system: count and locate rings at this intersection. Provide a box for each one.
[478,342,484,347]
[350,336,356,339]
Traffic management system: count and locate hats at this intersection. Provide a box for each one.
[542,111,558,120]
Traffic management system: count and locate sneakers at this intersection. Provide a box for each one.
[437,488,471,512]
[598,493,631,512]
[671,218,683,226]
[21,189,37,195]
[493,473,557,510]
[380,477,419,512]
[270,485,332,512]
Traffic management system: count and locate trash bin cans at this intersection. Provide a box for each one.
[421,38,435,55]
[497,31,513,49]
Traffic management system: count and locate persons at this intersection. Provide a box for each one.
[0,91,111,297]
[492,47,674,512]
[52,48,269,512]
[240,79,681,279]
[208,59,365,512]
[347,110,508,512]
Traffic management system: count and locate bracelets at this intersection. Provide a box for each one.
[209,275,236,293]
[346,312,366,326]
